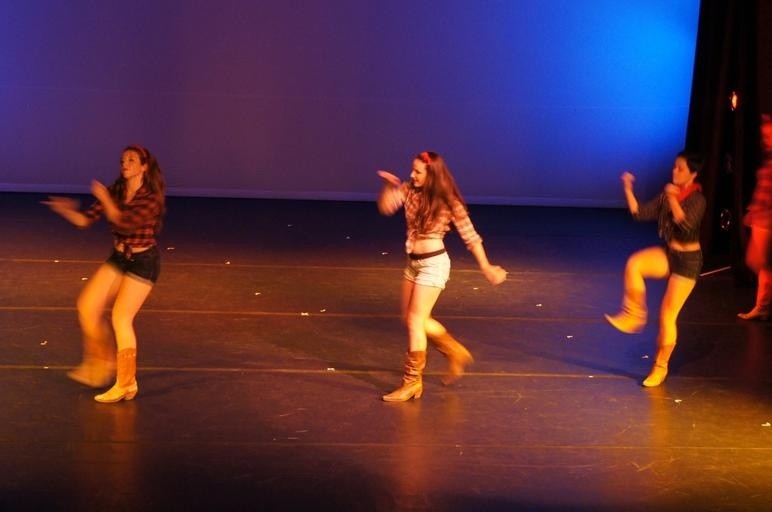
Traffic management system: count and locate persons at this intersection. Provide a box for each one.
[734,112,770,322]
[40,143,169,402]
[377,149,508,401]
[603,150,706,390]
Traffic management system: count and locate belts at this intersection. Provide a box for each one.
[410,248,446,260]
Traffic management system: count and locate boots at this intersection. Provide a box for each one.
[644,343,676,387]
[738,271,772,321]
[605,296,649,333]
[433,331,473,385]
[383,350,426,401]
[94,347,138,403]
